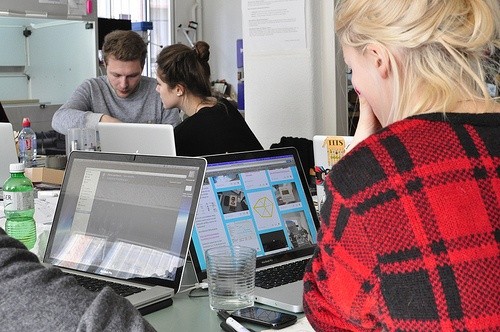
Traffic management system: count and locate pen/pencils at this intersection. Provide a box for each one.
[218,310,256,332]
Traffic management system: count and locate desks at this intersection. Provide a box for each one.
[141,259,305,332]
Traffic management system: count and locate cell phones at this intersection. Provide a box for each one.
[232,307,297,329]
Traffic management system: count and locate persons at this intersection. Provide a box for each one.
[51,30,185,134]
[302,0,500,332]
[153,41,265,166]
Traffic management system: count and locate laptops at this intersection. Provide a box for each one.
[0,123,19,189]
[189,147,321,313]
[313,136,354,215]
[98,123,176,156]
[43,150,207,309]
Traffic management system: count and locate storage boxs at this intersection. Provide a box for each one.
[24,168,64,185]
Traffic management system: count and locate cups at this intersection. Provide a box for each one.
[81,128,96,152]
[65,128,83,157]
[205,245,256,312]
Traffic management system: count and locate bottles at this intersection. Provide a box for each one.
[3,163,36,250]
[18,118,38,167]
